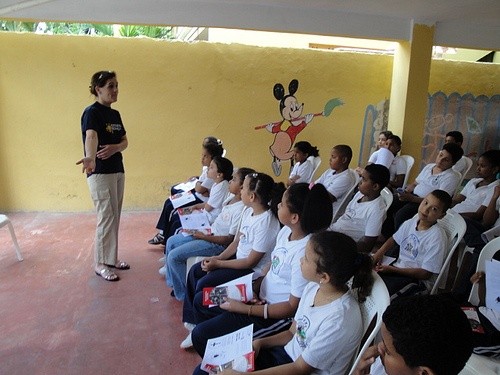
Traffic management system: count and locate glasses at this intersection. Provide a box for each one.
[98,70,114,80]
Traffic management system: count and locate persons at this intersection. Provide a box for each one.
[76,70,130,282]
[148,130,500,375]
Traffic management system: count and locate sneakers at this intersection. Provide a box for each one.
[148,233,165,245]
[180,321,197,349]
[159,256,167,274]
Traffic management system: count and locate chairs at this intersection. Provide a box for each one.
[186,155,500,375]
[0,214,24,261]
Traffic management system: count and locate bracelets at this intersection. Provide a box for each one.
[264,304,268,320]
[248,305,252,316]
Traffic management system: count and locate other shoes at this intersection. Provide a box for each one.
[170,289,175,298]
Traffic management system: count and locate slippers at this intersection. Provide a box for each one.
[112,259,131,270]
[95,266,119,281]
